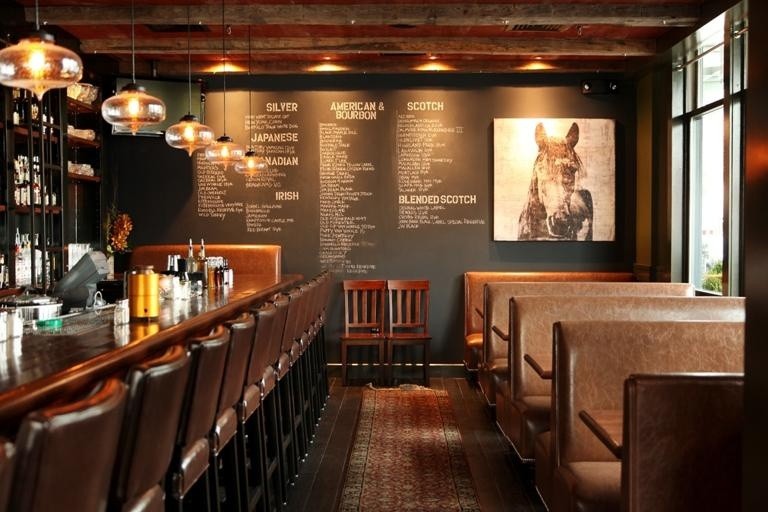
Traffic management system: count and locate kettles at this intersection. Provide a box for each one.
[124,266,160,322]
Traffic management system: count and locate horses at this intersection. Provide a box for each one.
[517,122,594,241]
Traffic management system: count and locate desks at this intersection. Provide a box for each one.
[0,273,306,419]
[577,406,624,490]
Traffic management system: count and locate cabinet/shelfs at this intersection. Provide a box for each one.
[1,87,100,292]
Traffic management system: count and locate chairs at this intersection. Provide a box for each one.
[0,440,16,512]
[386,278,431,385]
[278,269,333,473]
[214,312,257,512]
[171,324,232,512]
[244,300,279,512]
[116,344,193,512]
[340,280,387,384]
[15,377,130,511]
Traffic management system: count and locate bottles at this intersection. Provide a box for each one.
[114,324,130,346]
[113,300,126,325]
[196,237,206,272]
[123,298,130,322]
[6,309,24,337]
[185,238,195,273]
[208,265,233,288]
[15,227,56,287]
[208,286,231,309]
[11,89,54,134]
[0,314,7,343]
[12,155,57,207]
[0,254,9,290]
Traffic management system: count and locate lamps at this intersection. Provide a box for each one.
[206,1,246,171]
[165,1,215,155]
[236,0,268,177]
[102,2,167,134]
[0,1,84,100]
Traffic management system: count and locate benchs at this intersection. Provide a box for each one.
[506,294,746,461]
[482,282,696,407]
[550,320,746,509]
[623,373,746,511]
[463,270,636,387]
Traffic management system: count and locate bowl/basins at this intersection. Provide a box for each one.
[76,83,94,102]
[67,82,82,99]
[84,86,100,105]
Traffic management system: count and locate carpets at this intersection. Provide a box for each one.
[337,385,481,511]
